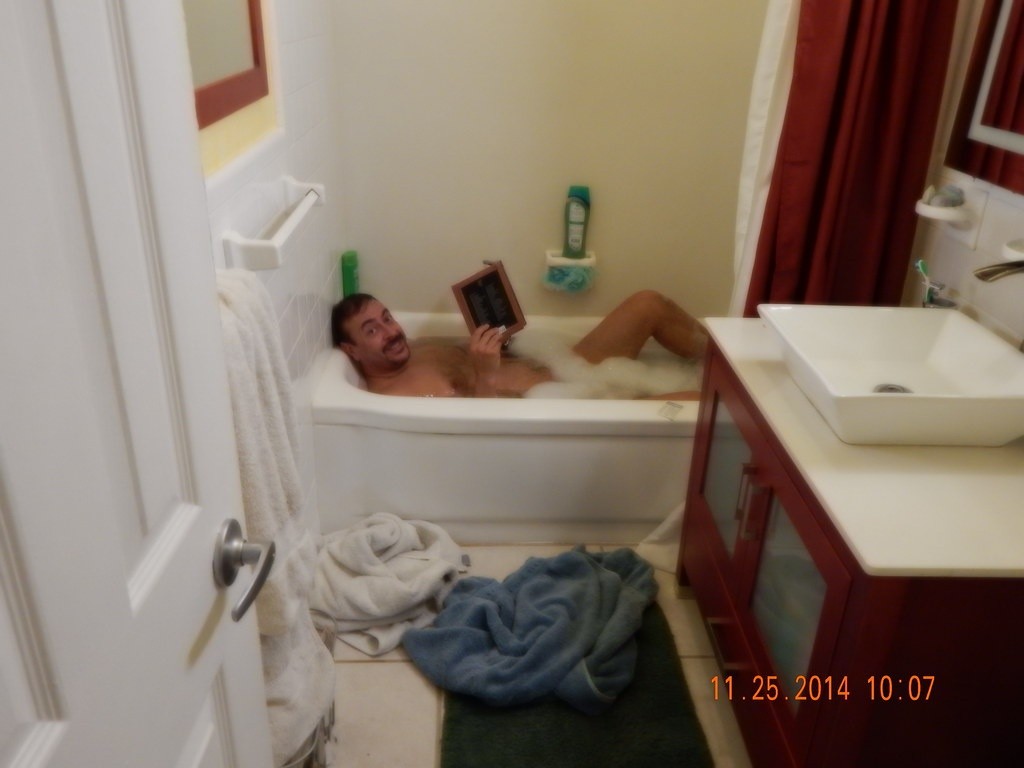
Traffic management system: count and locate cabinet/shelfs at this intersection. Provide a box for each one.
[669,314,1023,767]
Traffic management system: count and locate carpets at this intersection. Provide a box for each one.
[437,600,714,766]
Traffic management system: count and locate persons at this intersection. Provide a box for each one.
[330,292,713,401]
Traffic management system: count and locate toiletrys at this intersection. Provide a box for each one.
[562,185,593,260]
[342,249,359,299]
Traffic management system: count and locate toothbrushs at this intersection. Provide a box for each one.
[915,260,934,303]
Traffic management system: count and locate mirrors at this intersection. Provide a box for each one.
[180,0,272,130]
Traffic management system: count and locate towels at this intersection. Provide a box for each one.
[308,512,467,658]
[215,266,336,764]
[398,548,661,709]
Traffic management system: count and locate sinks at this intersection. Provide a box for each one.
[757,301,1024,445]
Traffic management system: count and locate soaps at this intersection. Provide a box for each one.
[921,185,962,209]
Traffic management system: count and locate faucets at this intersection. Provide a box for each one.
[973,261,1024,282]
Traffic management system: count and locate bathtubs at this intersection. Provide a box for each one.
[302,309,703,525]
